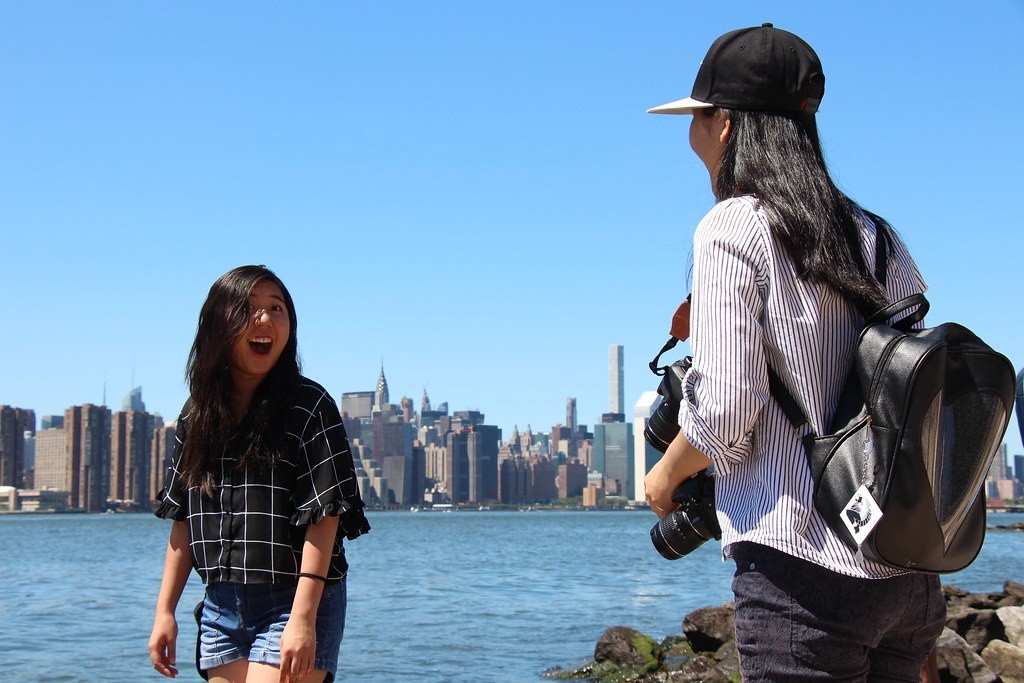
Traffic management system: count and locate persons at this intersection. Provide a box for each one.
[642,21,950,683]
[148,263,372,683]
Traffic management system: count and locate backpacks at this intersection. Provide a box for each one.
[766,209,1018,574]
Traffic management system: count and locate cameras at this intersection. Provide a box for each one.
[642,355,694,453]
[650,470,723,561]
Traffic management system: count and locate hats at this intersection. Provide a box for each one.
[646,22,825,119]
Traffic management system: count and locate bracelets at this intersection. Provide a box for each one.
[297,573,327,585]
